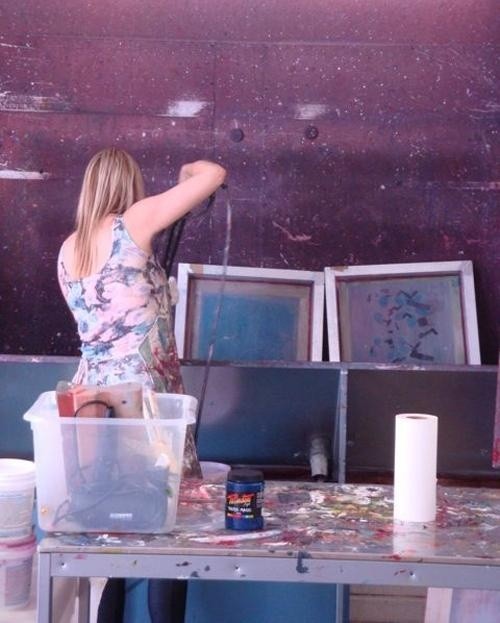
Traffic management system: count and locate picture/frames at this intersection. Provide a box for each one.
[324,261,481,367]
[173,262,324,363]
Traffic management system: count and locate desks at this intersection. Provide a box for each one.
[36,477,499,623]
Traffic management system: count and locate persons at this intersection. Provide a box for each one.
[54,145,229,623]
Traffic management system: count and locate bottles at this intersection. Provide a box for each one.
[224,469,265,531]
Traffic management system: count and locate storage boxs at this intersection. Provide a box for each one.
[23,391,198,535]
